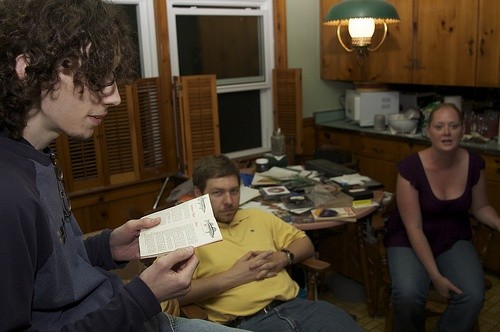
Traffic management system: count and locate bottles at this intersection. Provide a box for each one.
[270,130,286,156]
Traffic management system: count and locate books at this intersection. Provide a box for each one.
[311,207,357,223]
[137,194,224,260]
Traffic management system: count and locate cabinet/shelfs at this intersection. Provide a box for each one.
[318,0,500,87]
[317,125,500,271]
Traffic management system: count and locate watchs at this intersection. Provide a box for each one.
[280,249,295,267]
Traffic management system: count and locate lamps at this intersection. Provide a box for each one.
[323,0,400,51]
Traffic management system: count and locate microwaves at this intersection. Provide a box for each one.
[345,89,400,128]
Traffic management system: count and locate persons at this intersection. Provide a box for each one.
[384,103,500,332]
[0,1,254,332]
[175,154,366,332]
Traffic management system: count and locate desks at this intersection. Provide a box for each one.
[175,166,384,317]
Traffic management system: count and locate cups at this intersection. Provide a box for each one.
[256,159,270,171]
[374,114,387,133]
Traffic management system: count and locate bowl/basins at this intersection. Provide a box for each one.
[386,112,419,133]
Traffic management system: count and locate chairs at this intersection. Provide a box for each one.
[371,202,494,332]
[322,145,359,169]
[181,258,331,319]
